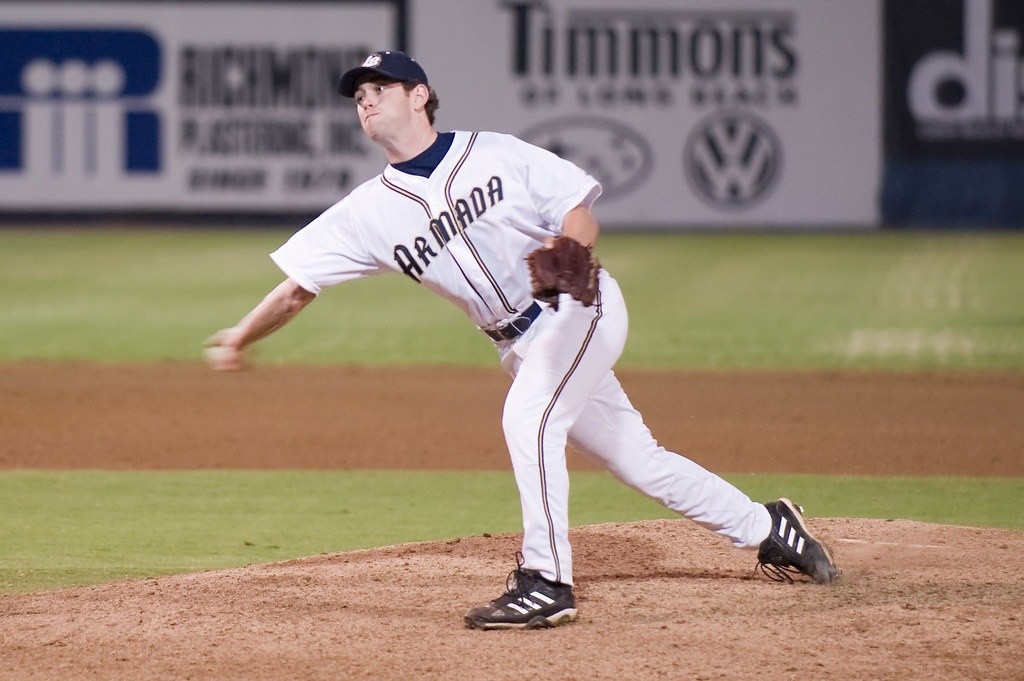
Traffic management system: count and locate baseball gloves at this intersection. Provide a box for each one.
[526,235,601,314]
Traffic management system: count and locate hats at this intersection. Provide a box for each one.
[337,49,427,98]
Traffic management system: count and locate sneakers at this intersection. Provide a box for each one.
[463,551,578,630]
[754,497,838,584]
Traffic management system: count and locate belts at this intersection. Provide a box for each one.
[485,301,542,342]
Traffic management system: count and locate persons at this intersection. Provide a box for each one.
[203,50,837,629]
